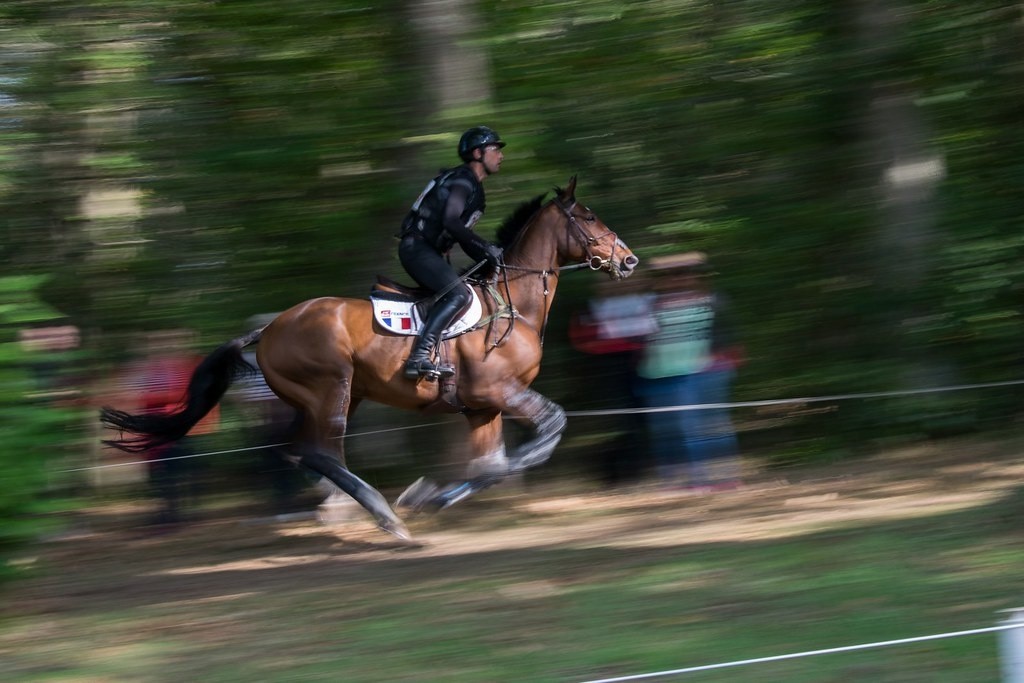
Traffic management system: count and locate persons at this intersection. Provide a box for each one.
[397,126,505,377]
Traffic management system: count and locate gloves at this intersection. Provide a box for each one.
[485,241,504,266]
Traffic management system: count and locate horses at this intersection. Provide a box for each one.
[98,174,639,541]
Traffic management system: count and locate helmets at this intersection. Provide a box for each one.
[458,125,506,156]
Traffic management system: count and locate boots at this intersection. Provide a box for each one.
[404,294,468,380]
